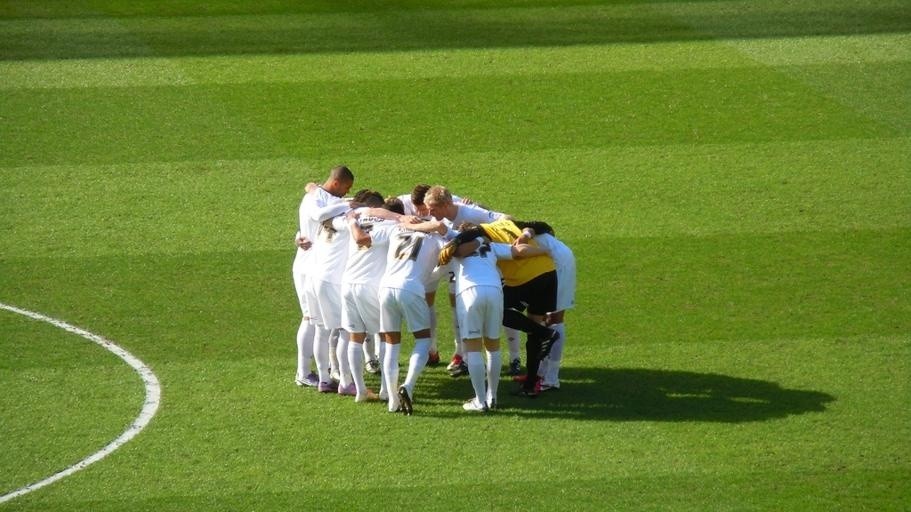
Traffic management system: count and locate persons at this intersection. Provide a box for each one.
[291,166,576,416]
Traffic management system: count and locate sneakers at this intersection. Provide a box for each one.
[462,396,498,412]
[540,328,560,360]
[446,354,468,377]
[295,370,413,416]
[506,359,561,396]
[364,360,381,373]
[426,351,440,366]
